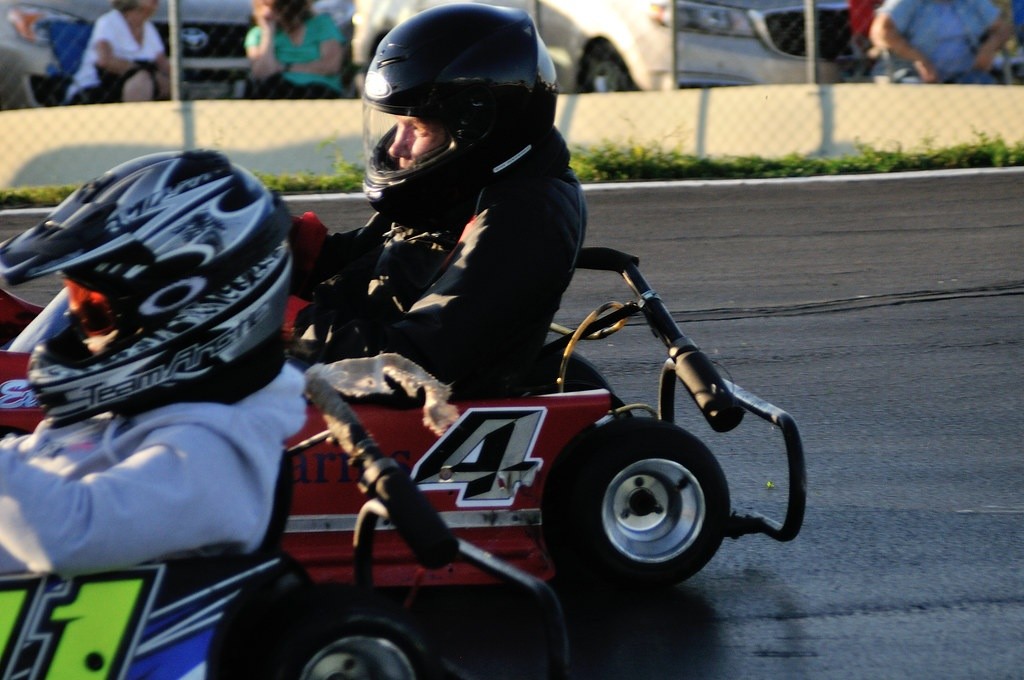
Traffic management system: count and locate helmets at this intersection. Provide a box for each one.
[362,3,559,225]
[0,149,293,431]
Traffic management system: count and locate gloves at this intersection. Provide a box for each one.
[289,212,326,293]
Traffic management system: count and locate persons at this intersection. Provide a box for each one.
[65,0,170,105]
[0,149,306,574]
[870,0,1012,83]
[263,3,588,403]
[245,0,345,100]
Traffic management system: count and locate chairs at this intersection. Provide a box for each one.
[38,19,94,104]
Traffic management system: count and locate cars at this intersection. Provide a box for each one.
[0,0,258,102]
[351,0,870,103]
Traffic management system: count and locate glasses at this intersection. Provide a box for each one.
[61,277,119,337]
